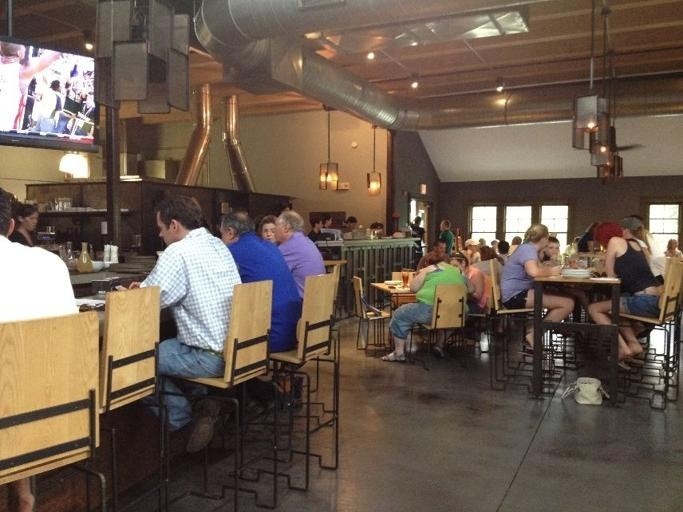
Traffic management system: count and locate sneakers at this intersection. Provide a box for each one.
[186,397,220,452]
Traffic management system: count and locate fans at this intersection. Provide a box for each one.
[602,125,643,152]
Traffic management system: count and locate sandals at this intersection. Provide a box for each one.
[381,350,407,363]
[433,346,444,359]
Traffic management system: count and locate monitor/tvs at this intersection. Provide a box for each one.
[0,35,99,153]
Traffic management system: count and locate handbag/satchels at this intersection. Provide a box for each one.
[574,377,603,405]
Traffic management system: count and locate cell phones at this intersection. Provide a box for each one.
[115,285,123,290]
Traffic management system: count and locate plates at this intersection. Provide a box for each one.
[562,273,590,279]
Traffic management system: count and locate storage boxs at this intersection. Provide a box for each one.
[405,75,419,89]
[142,159,180,181]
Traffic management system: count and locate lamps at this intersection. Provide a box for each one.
[495,77,503,93]
[366,124,382,199]
[572,0,623,183]
[319,104,337,191]
[82,30,94,51]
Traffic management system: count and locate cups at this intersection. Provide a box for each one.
[401,271,410,288]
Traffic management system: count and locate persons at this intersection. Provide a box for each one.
[382,215,509,362]
[220,208,326,425]
[0,189,81,510]
[7,204,39,248]
[501,218,682,370]
[1,42,94,137]
[117,195,242,452]
[307,214,384,242]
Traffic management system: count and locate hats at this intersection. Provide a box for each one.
[465,239,479,247]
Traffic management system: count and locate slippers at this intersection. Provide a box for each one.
[626,356,645,365]
[608,355,631,371]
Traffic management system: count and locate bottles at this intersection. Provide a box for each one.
[58,241,95,275]
[102,241,110,262]
[55,197,72,212]
[110,241,119,262]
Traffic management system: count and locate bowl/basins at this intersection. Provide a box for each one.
[91,261,104,272]
